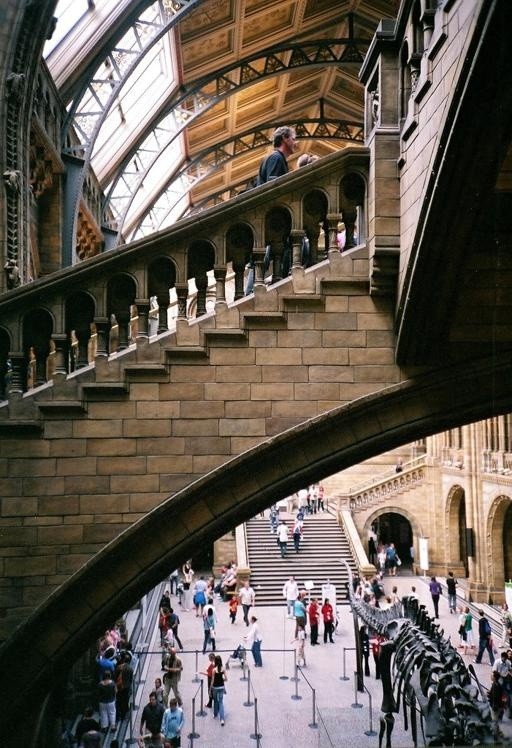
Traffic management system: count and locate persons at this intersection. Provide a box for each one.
[280,154,321,280]
[245,126,296,296]
[344,518,511,748]
[396,456,407,473]
[62,562,263,748]
[283,574,335,667]
[259,482,326,558]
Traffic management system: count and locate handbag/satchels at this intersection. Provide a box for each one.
[485,620,491,634]
